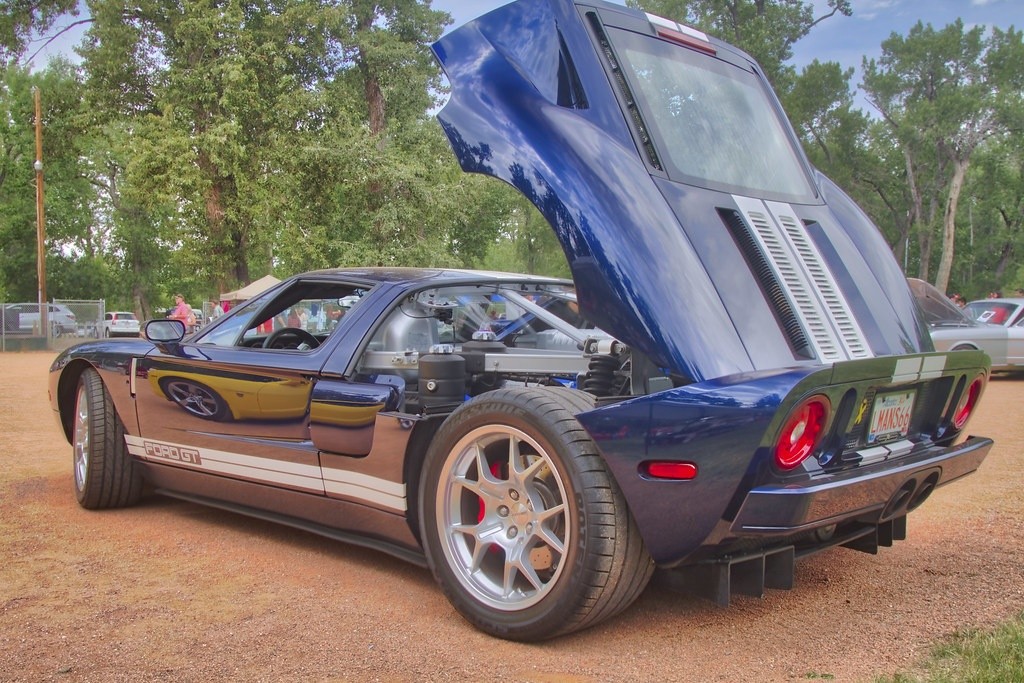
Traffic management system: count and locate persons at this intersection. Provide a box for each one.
[332,307,345,322]
[257,308,308,334]
[167,294,196,334]
[211,301,234,322]
[311,302,319,317]
[948,289,1024,308]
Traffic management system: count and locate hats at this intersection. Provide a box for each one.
[175,294,184,299]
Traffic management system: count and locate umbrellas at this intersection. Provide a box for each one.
[220,275,321,301]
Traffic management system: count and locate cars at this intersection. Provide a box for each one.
[92,311,139,338]
[46,1,997,644]
[906,277,1024,373]
[145,367,407,429]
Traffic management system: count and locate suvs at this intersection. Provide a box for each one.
[1,302,79,337]
